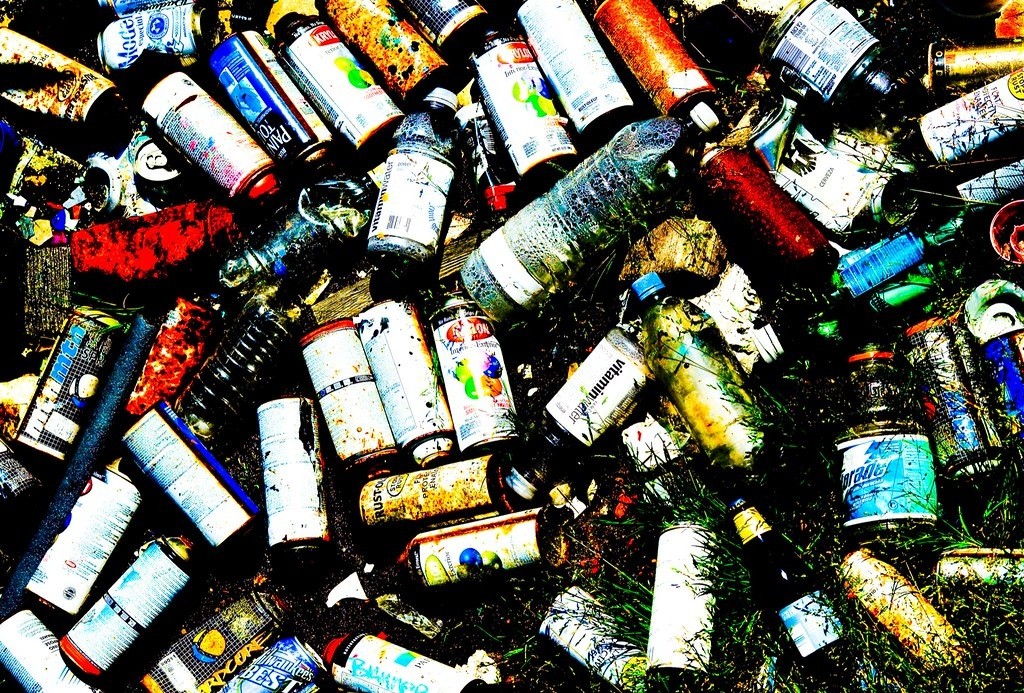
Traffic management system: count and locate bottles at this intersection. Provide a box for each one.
[0,0,1024,693]
